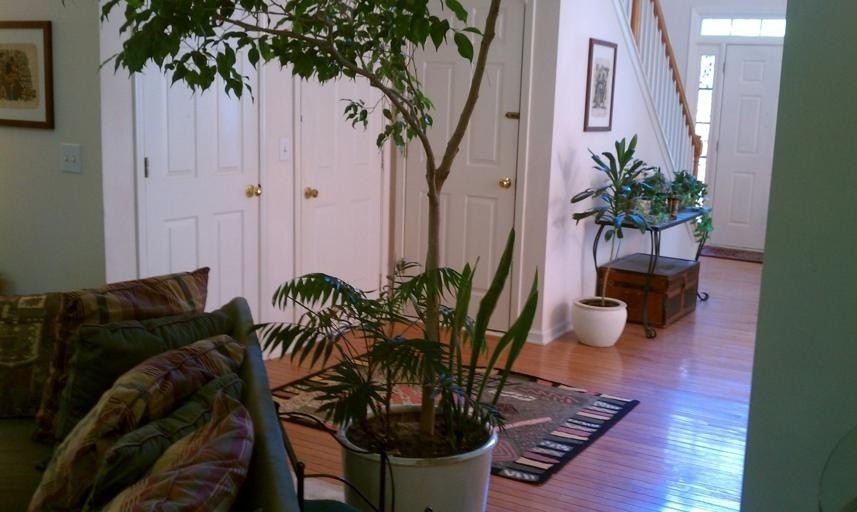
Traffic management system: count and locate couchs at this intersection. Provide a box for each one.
[0,296,303,511]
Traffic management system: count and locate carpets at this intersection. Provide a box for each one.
[267,334,640,488]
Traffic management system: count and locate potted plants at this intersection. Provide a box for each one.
[94,0,541,512]
[566,133,713,348]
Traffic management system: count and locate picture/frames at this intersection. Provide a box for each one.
[0,21,54,130]
[583,37,617,132]
